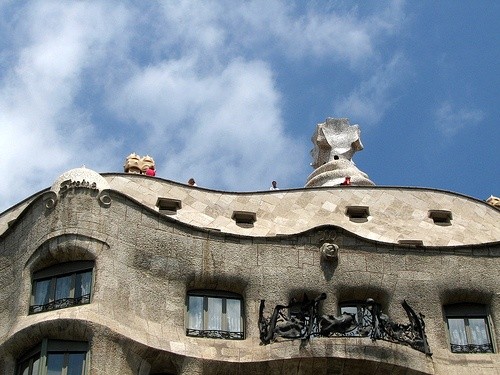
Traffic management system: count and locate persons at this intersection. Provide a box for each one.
[270,181,279,190]
[340,176,352,186]
[147,166,156,176]
[188,178,197,186]
